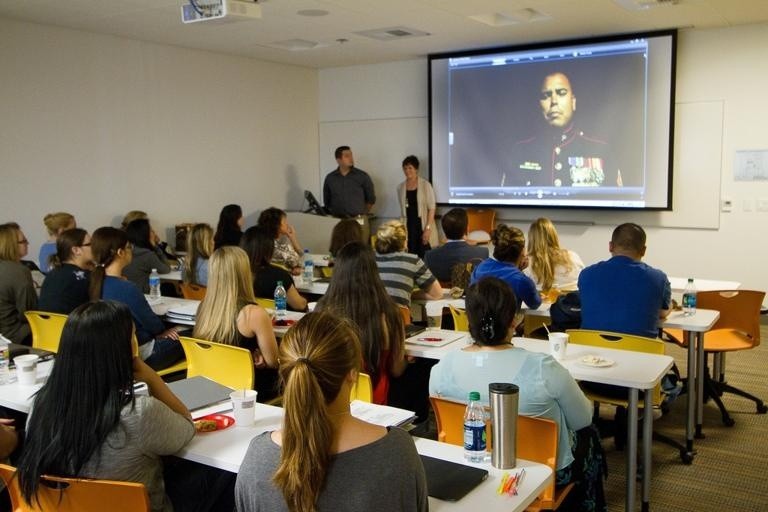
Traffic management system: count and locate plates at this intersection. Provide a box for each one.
[189,413,235,432]
[271,318,297,331]
[576,352,616,368]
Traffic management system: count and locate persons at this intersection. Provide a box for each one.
[365,220,442,345]
[122,210,174,258]
[215,205,244,251]
[427,209,488,292]
[184,224,215,288]
[15,301,193,511]
[121,219,183,298]
[428,279,607,510]
[39,228,93,315]
[399,157,438,252]
[579,224,679,449]
[322,147,378,243]
[523,217,582,314]
[242,227,307,312]
[194,246,284,398]
[86,227,194,368]
[259,208,304,273]
[329,218,362,258]
[233,315,427,511]
[40,212,77,274]
[313,246,413,406]
[0,223,41,351]
[468,226,542,334]
[497,71,622,188]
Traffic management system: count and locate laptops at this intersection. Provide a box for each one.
[164,375,238,413]
[419,453,489,504]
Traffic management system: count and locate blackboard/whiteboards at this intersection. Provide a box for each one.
[317,98,724,233]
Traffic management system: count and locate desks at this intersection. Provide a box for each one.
[0,351,551,512]
[158,271,720,464]
[671,279,742,384]
[143,294,674,512]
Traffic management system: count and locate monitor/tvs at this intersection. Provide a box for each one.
[302,190,326,215]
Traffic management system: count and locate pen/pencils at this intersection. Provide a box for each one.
[417,337,444,342]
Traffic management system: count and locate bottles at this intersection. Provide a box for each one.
[149,269,161,300]
[0,333,10,386]
[275,282,287,320]
[681,278,696,316]
[301,249,314,289]
[463,392,487,464]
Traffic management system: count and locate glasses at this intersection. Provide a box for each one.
[125,244,134,251]
[82,243,90,247]
[19,239,28,244]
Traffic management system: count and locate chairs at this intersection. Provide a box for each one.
[448,303,524,333]
[349,372,373,403]
[465,208,495,233]
[178,283,207,300]
[177,335,284,406]
[24,310,68,352]
[565,328,694,479]
[0,464,151,512]
[428,396,575,512]
[662,290,768,426]
[271,263,293,271]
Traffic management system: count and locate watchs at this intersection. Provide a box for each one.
[425,226,429,230]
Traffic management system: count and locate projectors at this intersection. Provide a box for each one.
[176,0,265,27]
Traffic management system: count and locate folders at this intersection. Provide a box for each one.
[167,303,199,320]
[136,375,238,410]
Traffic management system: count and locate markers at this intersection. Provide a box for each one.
[496,468,525,496]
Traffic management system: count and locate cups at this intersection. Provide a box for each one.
[230,389,257,427]
[13,354,39,387]
[307,301,318,313]
[425,301,444,337]
[548,331,569,361]
